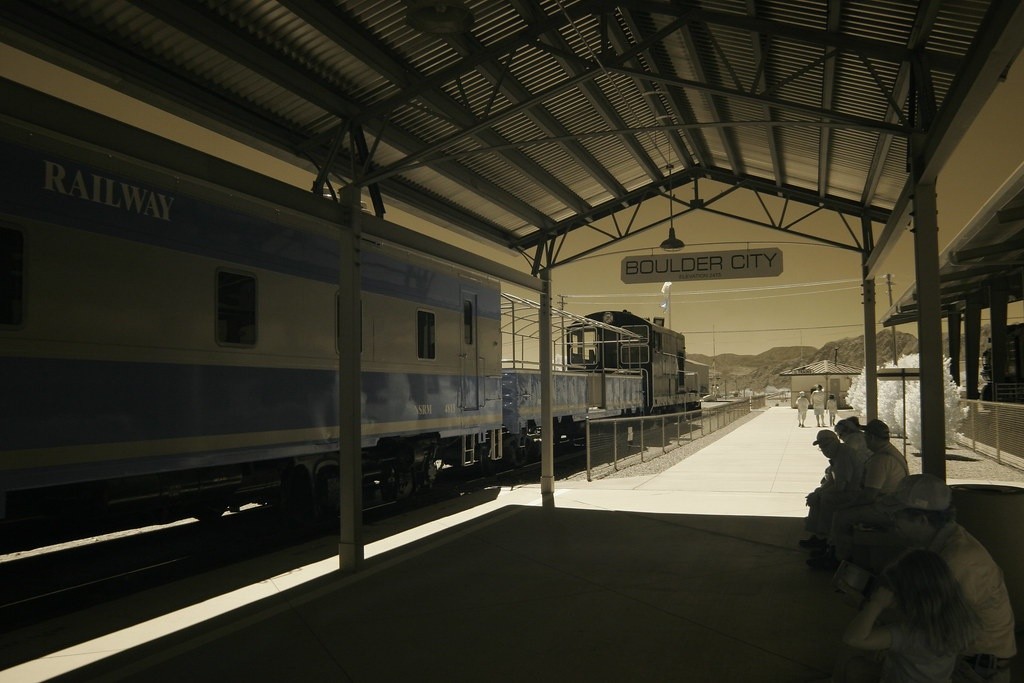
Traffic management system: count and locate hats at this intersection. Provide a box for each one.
[847,416,865,430]
[860,418,889,438]
[882,474,953,512]
[812,430,837,446]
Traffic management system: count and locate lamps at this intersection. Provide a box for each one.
[406,0,476,35]
[660,163,685,252]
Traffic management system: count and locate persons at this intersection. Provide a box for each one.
[799,416,1018,683]
[796,384,838,427]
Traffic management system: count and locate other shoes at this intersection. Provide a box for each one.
[807,546,837,572]
[810,548,826,558]
[800,535,826,548]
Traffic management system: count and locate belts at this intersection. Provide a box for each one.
[959,653,1012,669]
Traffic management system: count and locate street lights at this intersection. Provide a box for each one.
[882,271,899,364]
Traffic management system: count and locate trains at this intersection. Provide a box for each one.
[0,119,704,615]
[978,323,1024,402]
